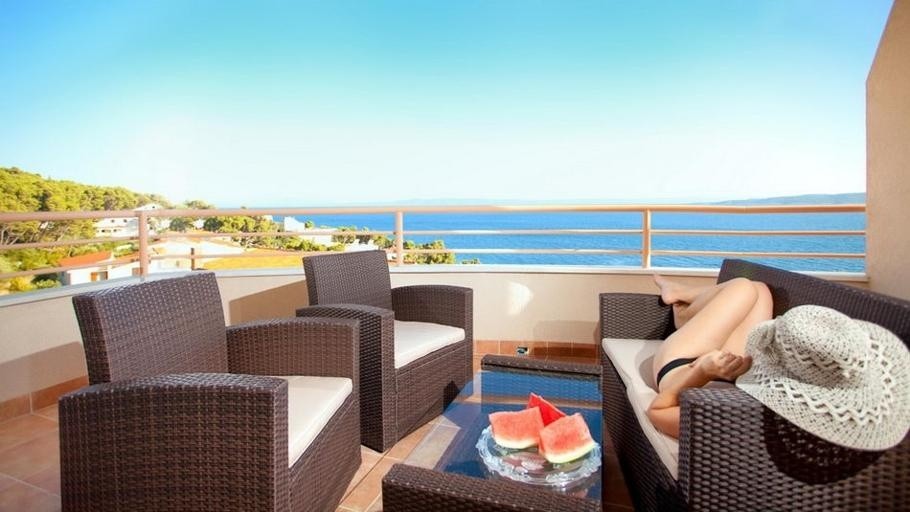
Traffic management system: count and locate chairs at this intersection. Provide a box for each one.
[296,249,473,455]
[57,268,361,511]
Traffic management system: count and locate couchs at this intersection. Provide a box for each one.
[598,258,910,511]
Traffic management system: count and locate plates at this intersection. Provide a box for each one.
[475,423,602,489]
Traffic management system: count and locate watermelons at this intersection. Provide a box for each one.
[538,413,595,462]
[488,406,543,449]
[524,392,565,428]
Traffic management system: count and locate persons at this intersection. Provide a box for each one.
[645,272,909,451]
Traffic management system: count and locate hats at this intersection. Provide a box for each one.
[735,305,910,452]
